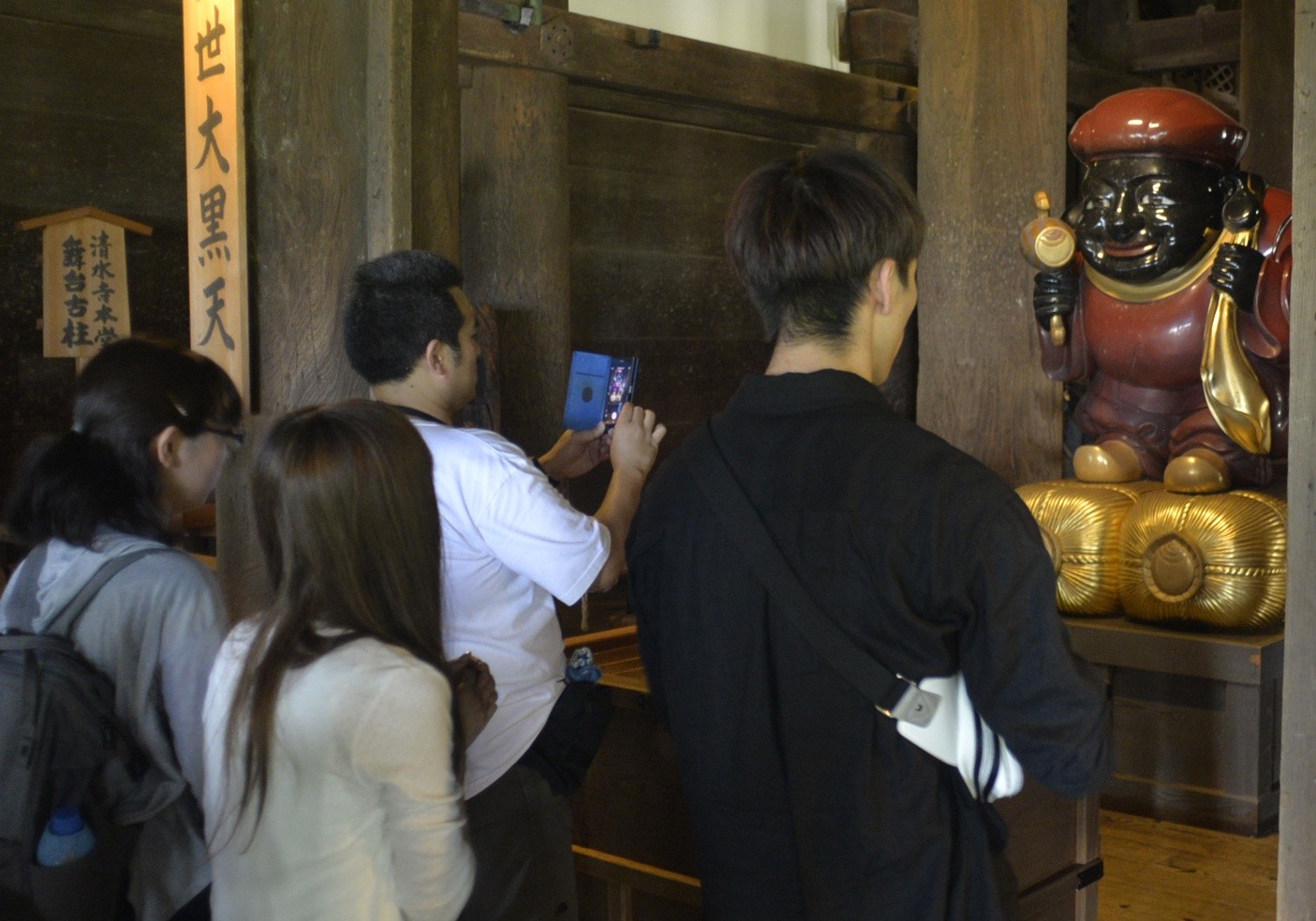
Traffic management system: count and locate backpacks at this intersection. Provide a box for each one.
[0,547,189,920]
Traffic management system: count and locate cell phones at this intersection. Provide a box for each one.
[602,354,637,427]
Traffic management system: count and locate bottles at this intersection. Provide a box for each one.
[35,809,94,865]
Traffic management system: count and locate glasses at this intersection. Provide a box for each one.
[193,425,245,450]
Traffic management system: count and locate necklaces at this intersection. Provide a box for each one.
[396,405,446,424]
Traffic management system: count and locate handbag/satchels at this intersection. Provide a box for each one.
[890,671,1025,801]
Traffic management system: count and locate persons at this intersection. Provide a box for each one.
[627,145,1111,921]
[201,400,500,921]
[342,252,669,921]
[0,337,248,921]
[1033,87,1293,494]
